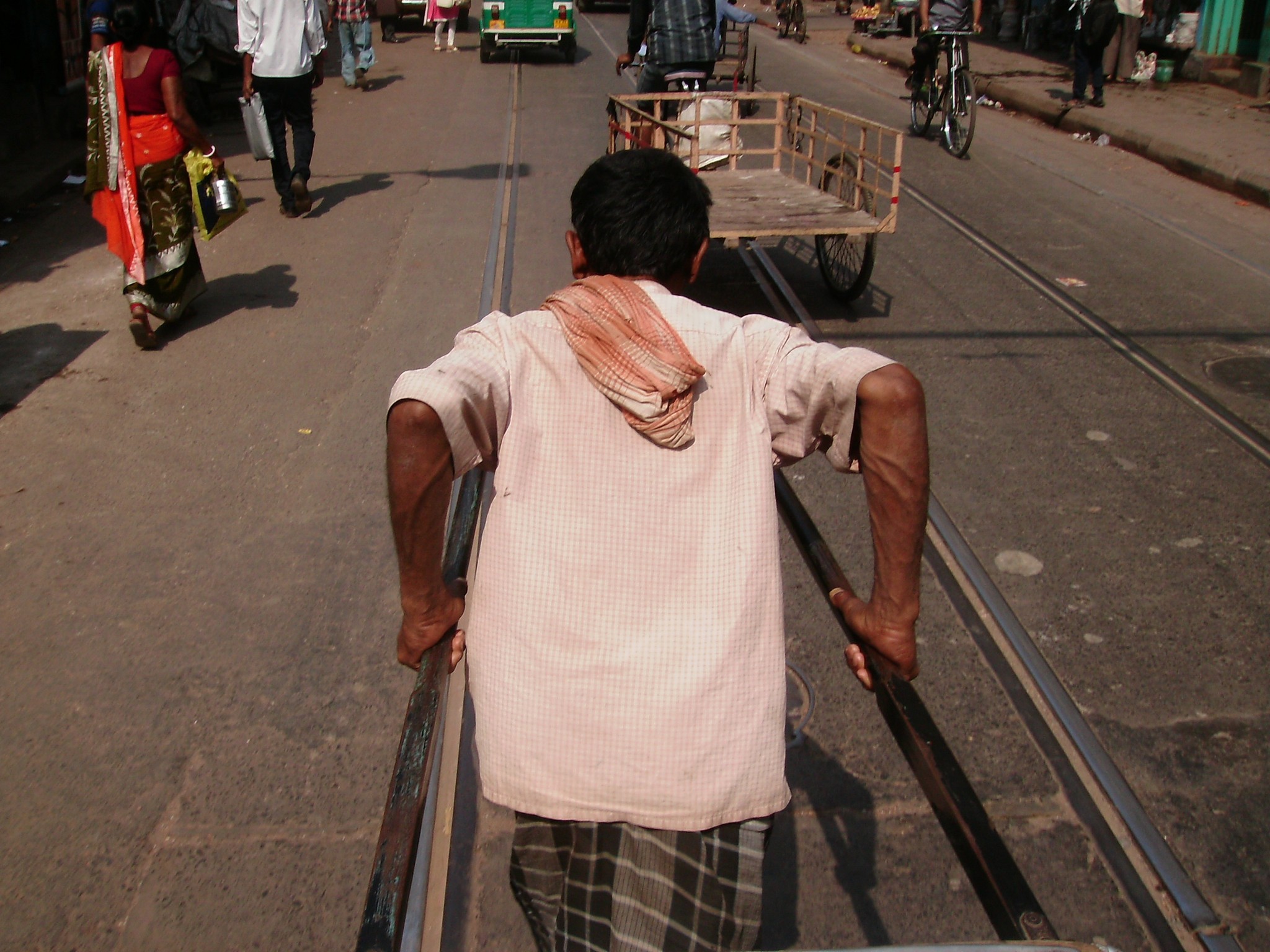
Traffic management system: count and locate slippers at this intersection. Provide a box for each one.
[129,319,157,347]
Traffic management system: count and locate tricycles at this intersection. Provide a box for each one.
[636,16,762,115]
[608,45,903,307]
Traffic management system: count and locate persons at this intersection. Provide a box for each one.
[232,0,329,217]
[83,0,225,351]
[775,0,802,18]
[326,0,382,91]
[862,0,875,7]
[1067,0,1118,108]
[905,0,985,131]
[613,0,757,117]
[426,0,462,52]
[380,144,934,952]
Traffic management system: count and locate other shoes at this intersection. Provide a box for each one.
[354,68,369,89]
[382,34,402,43]
[291,174,312,212]
[905,72,924,91]
[278,202,298,217]
[434,44,458,53]
[1056,99,1105,111]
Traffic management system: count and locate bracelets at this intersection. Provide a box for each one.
[202,144,216,158]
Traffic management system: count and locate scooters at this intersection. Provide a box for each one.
[479,0,576,62]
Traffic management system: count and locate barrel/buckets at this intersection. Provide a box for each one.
[212,178,239,213]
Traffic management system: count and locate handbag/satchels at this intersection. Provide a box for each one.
[239,91,275,162]
[184,146,250,243]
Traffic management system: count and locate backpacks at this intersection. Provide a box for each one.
[1078,0,1119,49]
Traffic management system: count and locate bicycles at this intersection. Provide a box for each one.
[900,22,982,159]
[774,0,807,44]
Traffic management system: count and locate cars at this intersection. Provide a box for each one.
[397,0,472,34]
